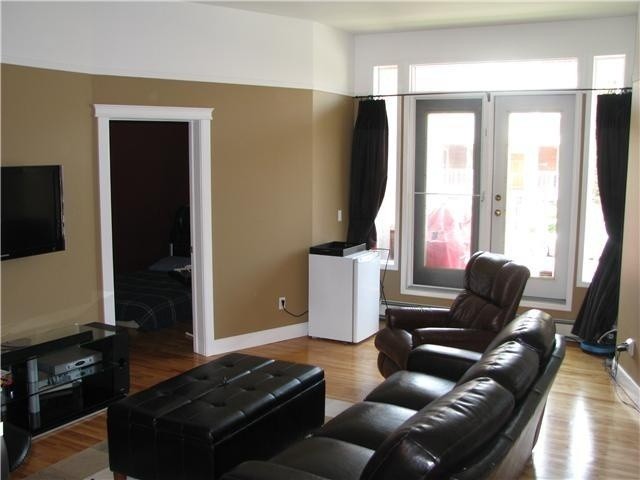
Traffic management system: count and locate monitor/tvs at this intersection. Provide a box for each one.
[0,163,66,263]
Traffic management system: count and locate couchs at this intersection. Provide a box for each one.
[221,307,565,480]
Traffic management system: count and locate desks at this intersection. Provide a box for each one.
[0,321,140,441]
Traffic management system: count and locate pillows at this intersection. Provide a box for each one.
[148,254,192,275]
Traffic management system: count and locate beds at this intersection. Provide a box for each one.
[116,242,192,336]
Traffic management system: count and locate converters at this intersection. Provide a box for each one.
[617,345,626,351]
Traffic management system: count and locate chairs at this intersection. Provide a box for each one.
[374,251,532,379]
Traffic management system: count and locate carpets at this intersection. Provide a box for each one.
[20,397,356,480]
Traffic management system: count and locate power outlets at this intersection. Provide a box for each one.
[278,297,286,310]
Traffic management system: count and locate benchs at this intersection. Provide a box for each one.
[106,350,326,480]
